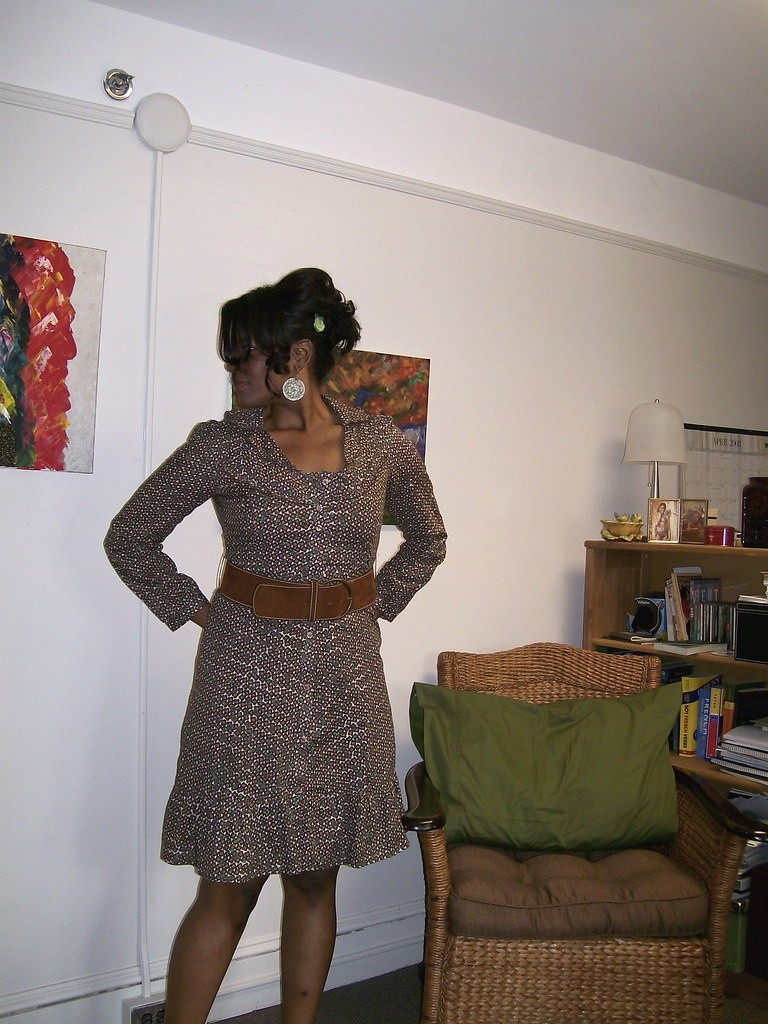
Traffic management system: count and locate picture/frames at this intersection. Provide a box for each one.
[679,498,708,544]
[673,572,702,640]
[647,499,681,544]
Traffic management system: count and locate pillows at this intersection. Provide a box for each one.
[407,681,682,853]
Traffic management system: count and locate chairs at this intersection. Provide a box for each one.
[400,642,768,1024]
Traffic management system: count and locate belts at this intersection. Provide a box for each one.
[217,559,375,621]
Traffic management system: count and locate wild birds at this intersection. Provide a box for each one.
[614,511,642,523]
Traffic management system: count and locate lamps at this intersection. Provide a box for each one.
[622,400,688,497]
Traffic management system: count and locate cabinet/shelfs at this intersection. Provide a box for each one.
[583,542,768,795]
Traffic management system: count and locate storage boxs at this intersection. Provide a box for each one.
[725,866,768,981]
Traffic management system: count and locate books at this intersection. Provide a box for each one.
[660,660,768,899]
[666,566,768,654]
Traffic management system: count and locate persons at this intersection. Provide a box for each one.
[654,503,672,542]
[103,267,448,1023]
